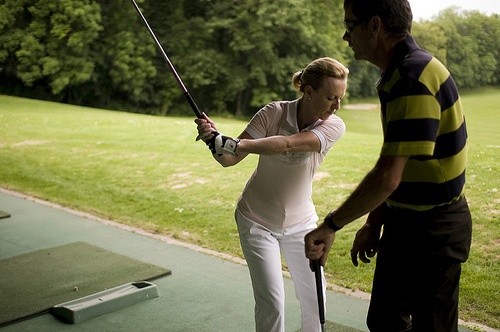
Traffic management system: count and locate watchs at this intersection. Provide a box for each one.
[323,208,345,231]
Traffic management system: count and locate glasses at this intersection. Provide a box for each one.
[342,19,369,35]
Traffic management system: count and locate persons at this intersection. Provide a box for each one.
[304,0,473,332]
[194,57,350,332]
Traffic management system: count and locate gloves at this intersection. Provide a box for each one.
[195,132,239,159]
